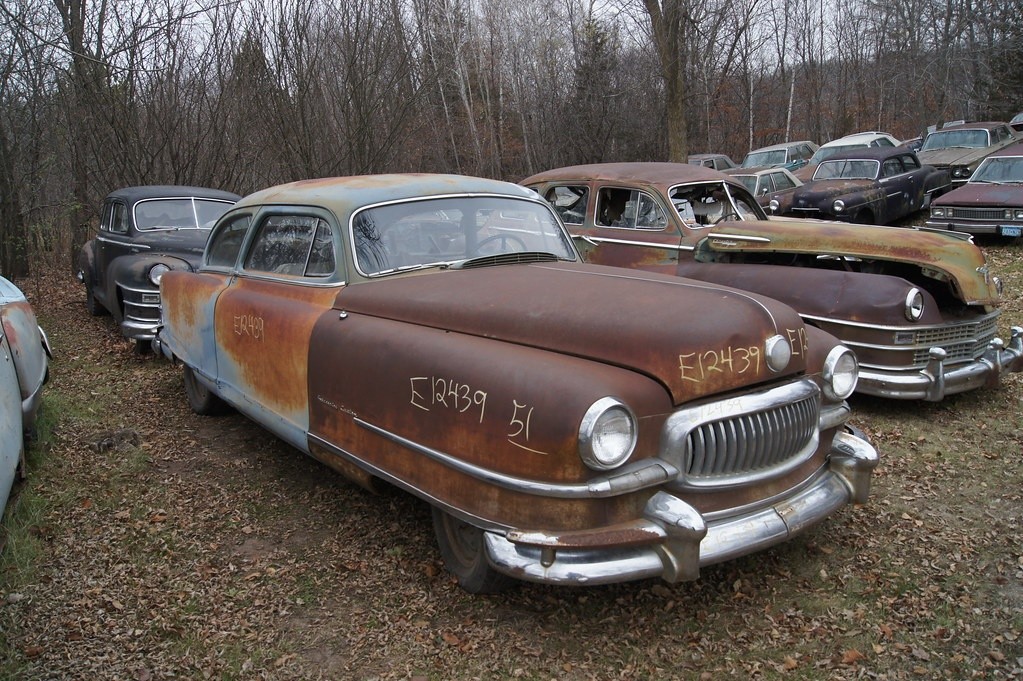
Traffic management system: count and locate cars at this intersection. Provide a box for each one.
[77,184,250,355]
[435,160,1023,402]
[768,147,952,226]
[925,143,1023,236]
[0,275,53,525]
[913,121,1023,190]
[154,174,881,599]
[667,140,821,225]
[807,130,924,177]
